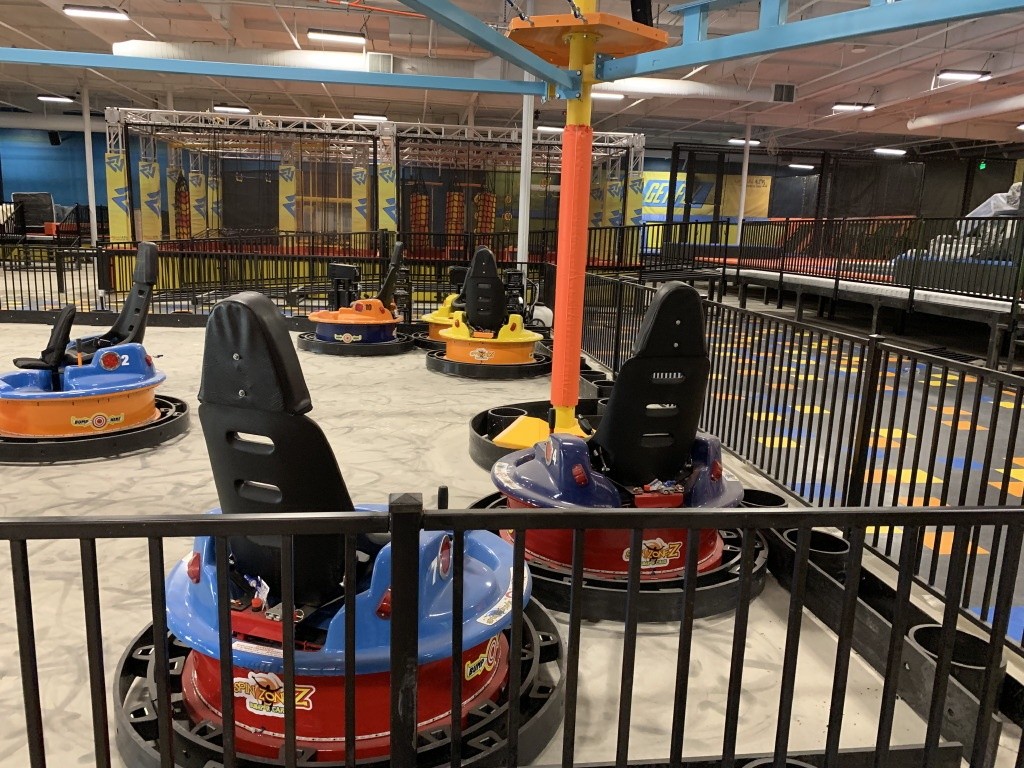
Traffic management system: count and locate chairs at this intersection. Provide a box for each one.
[451,244,507,332]
[198,290,390,647]
[377,240,404,306]
[580,280,711,486]
[13,242,159,391]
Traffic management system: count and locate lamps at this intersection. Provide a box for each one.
[353,113,387,121]
[831,102,875,112]
[307,28,366,43]
[214,103,249,113]
[62,4,129,20]
[935,23,991,81]
